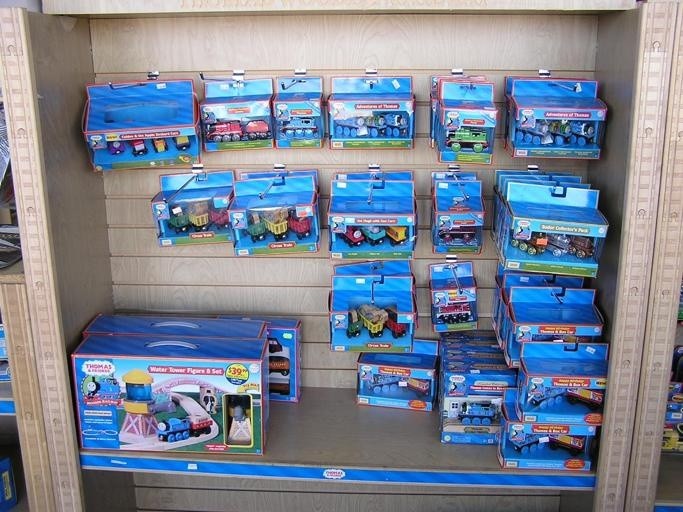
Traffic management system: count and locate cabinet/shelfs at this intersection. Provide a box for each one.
[2,1,683,511]
